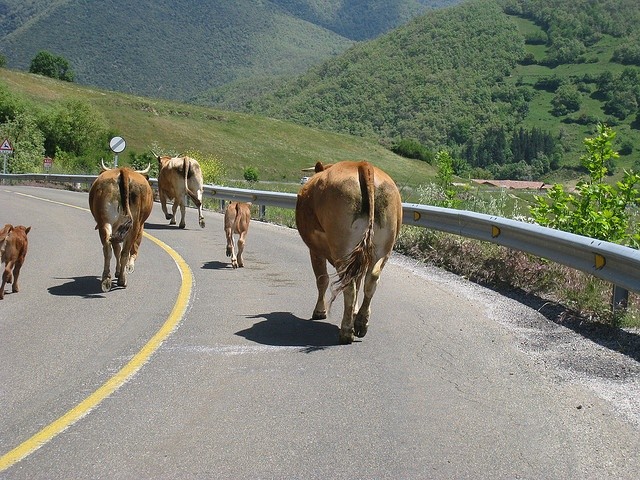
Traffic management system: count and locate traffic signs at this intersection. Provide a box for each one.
[44,158,53,168]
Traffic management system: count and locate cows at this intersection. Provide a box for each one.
[149,148,205,229]
[224,200,252,270]
[0,223,31,301]
[295,159,404,345]
[88,157,155,293]
